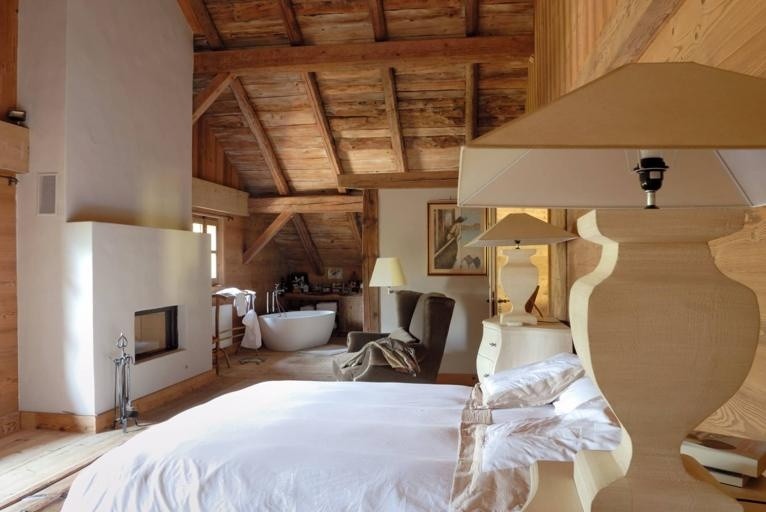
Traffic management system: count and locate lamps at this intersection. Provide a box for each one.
[462,212,580,326]
[368,255,407,297]
[453,58,766,511]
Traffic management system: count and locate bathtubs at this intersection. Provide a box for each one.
[257,309,337,352]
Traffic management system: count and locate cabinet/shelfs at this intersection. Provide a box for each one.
[337,292,365,338]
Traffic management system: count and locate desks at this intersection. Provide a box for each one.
[211,290,261,377]
[274,291,340,338]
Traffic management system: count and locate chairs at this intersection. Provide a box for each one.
[330,287,455,384]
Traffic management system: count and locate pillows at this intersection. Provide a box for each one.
[476,352,585,401]
[472,413,626,474]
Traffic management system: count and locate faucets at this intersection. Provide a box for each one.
[270,288,286,312]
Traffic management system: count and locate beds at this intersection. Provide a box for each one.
[60,379,622,512]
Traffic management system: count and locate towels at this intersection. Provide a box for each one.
[215,286,262,350]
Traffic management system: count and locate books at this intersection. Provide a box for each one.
[682,433,766,504]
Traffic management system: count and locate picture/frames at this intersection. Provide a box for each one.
[426,201,489,277]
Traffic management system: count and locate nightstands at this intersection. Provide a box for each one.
[513,452,726,511]
[473,314,575,384]
